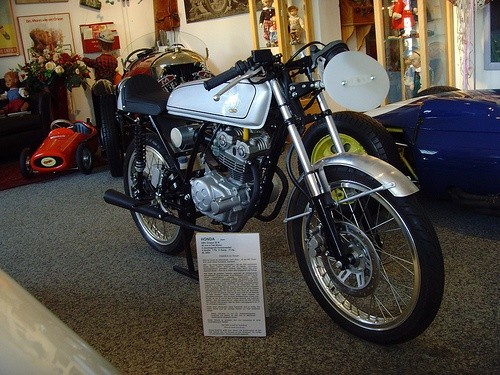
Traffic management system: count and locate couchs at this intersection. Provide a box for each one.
[0,79,50,165]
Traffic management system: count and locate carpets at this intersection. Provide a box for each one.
[0,152,108,191]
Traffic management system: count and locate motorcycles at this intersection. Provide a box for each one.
[103,39,445,347]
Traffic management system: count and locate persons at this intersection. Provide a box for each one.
[287,5,305,45]
[80,31,118,156]
[0,72,29,115]
[259,0,275,47]
[387,0,418,37]
[402,49,434,99]
[269,17,278,47]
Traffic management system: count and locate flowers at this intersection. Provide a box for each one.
[12,31,91,98]
